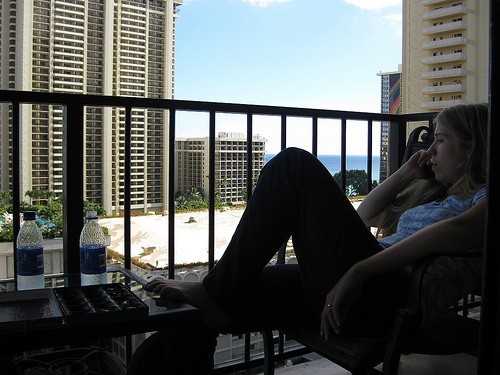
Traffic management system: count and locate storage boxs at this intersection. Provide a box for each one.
[0,283,150,335]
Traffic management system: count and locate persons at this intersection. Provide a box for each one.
[142,102,489,375]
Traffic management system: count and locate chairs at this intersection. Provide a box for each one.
[262,126,483,375]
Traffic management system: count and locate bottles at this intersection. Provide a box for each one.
[80,211,107,286]
[17,211,45,290]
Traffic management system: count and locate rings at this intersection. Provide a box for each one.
[327,304,332,310]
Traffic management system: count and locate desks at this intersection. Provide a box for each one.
[0,268,200,375]
